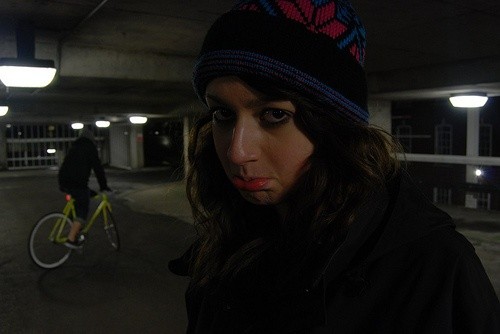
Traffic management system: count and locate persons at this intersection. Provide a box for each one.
[57,125,113,250]
[167,0,500,334]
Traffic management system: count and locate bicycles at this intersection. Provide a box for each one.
[28,188,121,271]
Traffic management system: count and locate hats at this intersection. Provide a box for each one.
[79,123,97,143]
[191,0,369,127]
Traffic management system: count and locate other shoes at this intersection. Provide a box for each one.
[64,238,82,249]
